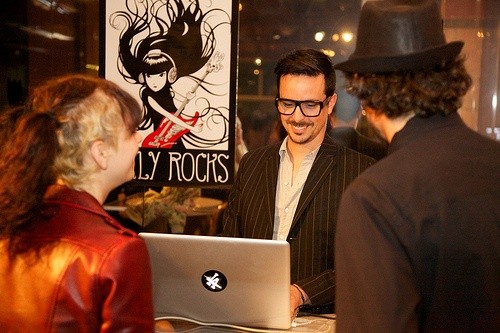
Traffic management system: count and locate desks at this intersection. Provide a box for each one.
[105,196,227,233]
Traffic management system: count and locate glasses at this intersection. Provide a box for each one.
[274,95,331,116]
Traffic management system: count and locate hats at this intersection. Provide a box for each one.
[333,0,464,74]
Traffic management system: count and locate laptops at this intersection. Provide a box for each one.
[138,232,293,330]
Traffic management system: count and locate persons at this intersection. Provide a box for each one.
[0,75,386,333]
[329,0,500,333]
[213,48,383,324]
